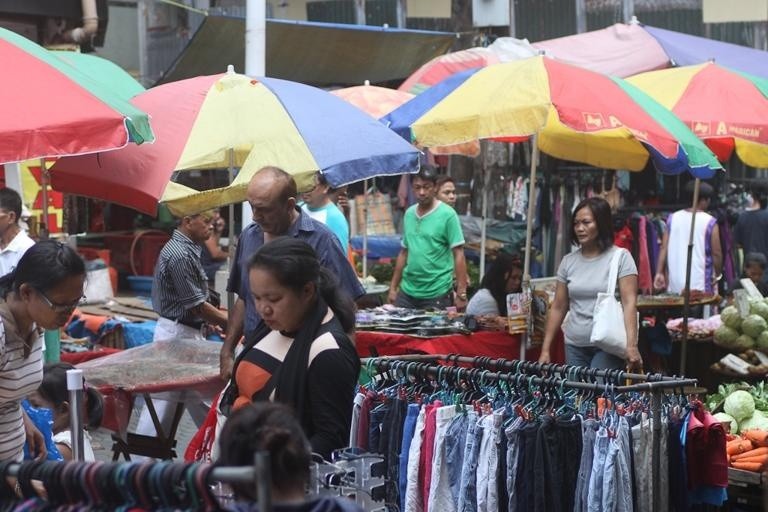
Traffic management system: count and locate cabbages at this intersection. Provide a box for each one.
[712,390,768,441]
[714,296,768,348]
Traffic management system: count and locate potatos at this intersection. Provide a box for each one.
[711,349,767,378]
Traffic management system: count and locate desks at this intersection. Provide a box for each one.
[354,327,565,367]
[350,213,542,287]
[72,335,232,462]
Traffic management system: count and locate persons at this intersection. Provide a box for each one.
[1,240,86,512]
[219,400,365,511]
[184,236,360,493]
[0,163,767,377]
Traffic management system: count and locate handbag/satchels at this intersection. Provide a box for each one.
[590,292,639,358]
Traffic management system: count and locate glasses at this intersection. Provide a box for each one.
[36,290,88,313]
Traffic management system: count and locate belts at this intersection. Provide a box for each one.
[163,315,202,329]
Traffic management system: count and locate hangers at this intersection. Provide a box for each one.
[0,460,233,512]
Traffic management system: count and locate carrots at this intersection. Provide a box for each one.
[725,428,768,473]
